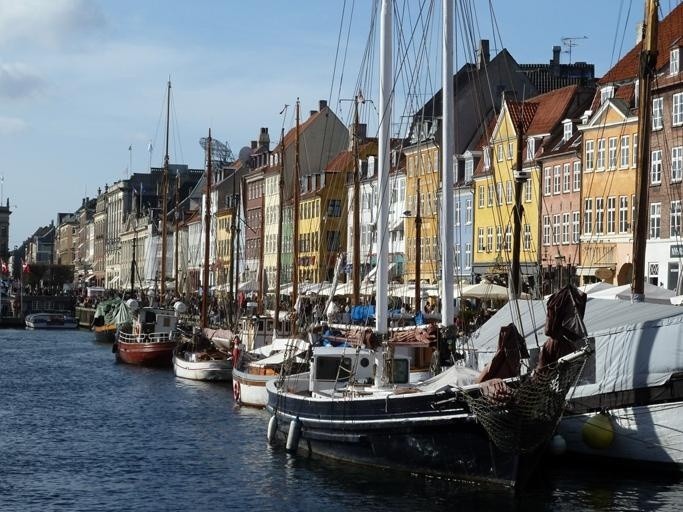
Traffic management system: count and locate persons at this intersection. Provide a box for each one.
[209,278,530,309]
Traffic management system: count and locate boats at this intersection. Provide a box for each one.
[23,311,80,331]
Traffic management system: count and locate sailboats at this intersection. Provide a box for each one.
[90,1,683,489]
